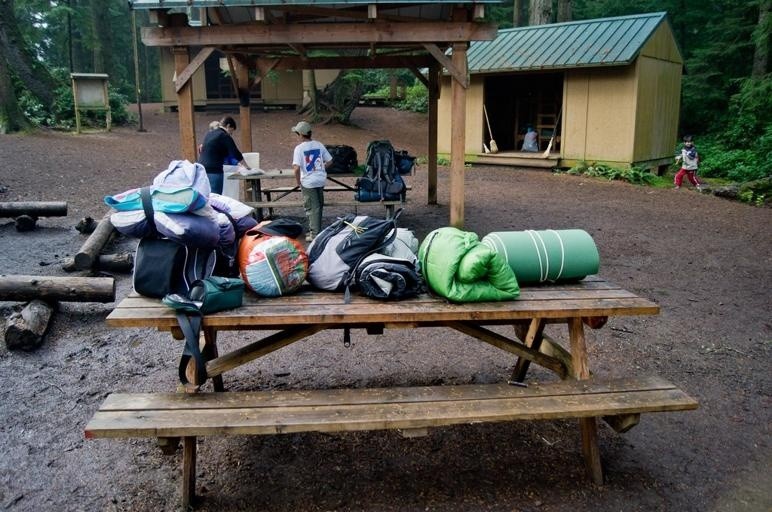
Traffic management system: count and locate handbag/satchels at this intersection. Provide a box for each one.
[132,235,245,316]
[323,144,358,174]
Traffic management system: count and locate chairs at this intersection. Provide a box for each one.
[512,111,557,153]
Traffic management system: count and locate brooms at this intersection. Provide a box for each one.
[543,107,562,161]
[484,105,499,154]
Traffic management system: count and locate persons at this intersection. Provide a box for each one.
[673,135,702,192]
[198,121,240,165]
[291,121,333,242]
[196,115,251,196]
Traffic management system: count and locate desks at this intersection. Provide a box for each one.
[106,267,661,454]
[226,164,413,220]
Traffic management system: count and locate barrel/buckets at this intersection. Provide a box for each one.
[241,152,259,170]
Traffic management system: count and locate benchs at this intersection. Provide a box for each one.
[244,182,412,214]
[84,375,698,508]
[241,199,403,229]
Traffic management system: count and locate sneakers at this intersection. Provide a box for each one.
[306,231,318,241]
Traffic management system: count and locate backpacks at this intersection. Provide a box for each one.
[306,207,406,290]
[365,139,398,185]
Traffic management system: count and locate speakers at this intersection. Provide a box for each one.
[188,7,207,26]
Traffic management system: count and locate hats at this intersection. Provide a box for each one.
[291,121,312,136]
[246,218,302,239]
